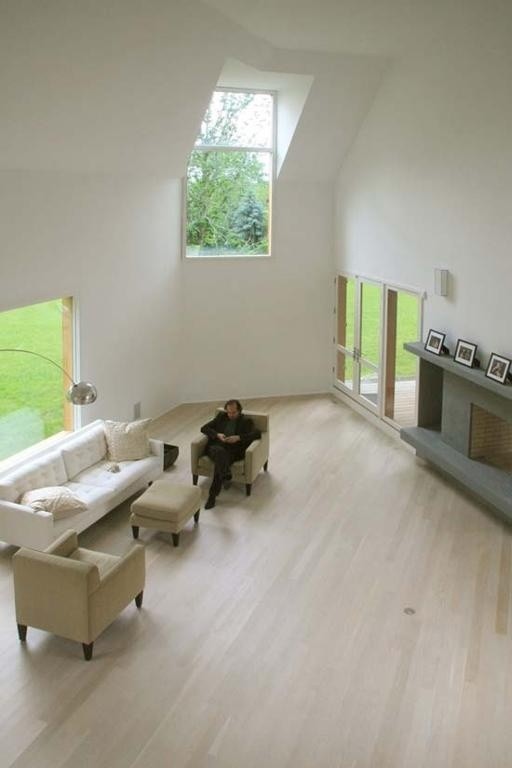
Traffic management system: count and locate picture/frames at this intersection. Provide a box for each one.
[421,328,511,388]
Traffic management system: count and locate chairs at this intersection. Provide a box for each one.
[189,408,271,498]
[14,528,148,661]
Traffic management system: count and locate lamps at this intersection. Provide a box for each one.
[0,346,98,408]
[432,266,449,297]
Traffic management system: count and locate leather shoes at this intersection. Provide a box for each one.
[224,482,231,489]
[205,496,215,509]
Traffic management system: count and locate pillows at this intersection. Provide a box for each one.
[103,419,153,462]
[21,485,89,521]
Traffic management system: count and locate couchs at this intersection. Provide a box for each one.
[1,419,165,551]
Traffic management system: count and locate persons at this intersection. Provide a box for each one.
[199,398,260,510]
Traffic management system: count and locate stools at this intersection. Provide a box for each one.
[131,479,203,547]
